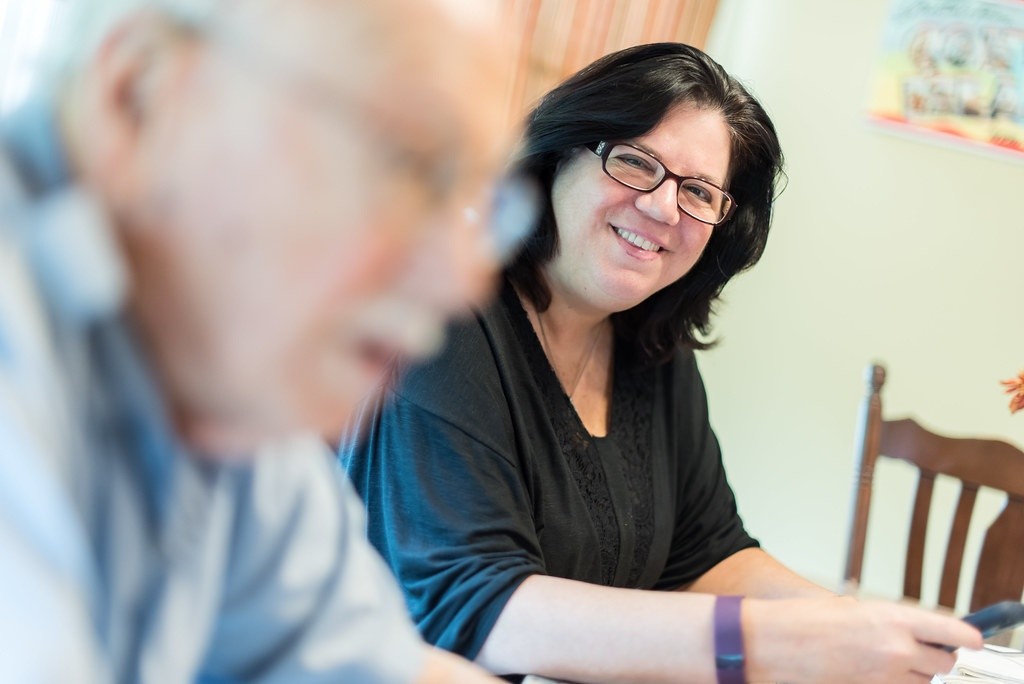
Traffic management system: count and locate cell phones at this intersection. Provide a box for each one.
[926,601,1024,654]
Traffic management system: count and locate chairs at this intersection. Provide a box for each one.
[843,361,1024,649]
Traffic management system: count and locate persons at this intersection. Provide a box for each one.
[0,2,516,684]
[337,44,987,684]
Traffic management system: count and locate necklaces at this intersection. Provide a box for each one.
[538,304,606,401]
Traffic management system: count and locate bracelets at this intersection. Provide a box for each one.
[714,594,750,684]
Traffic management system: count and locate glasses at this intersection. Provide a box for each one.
[583,140,738,226]
